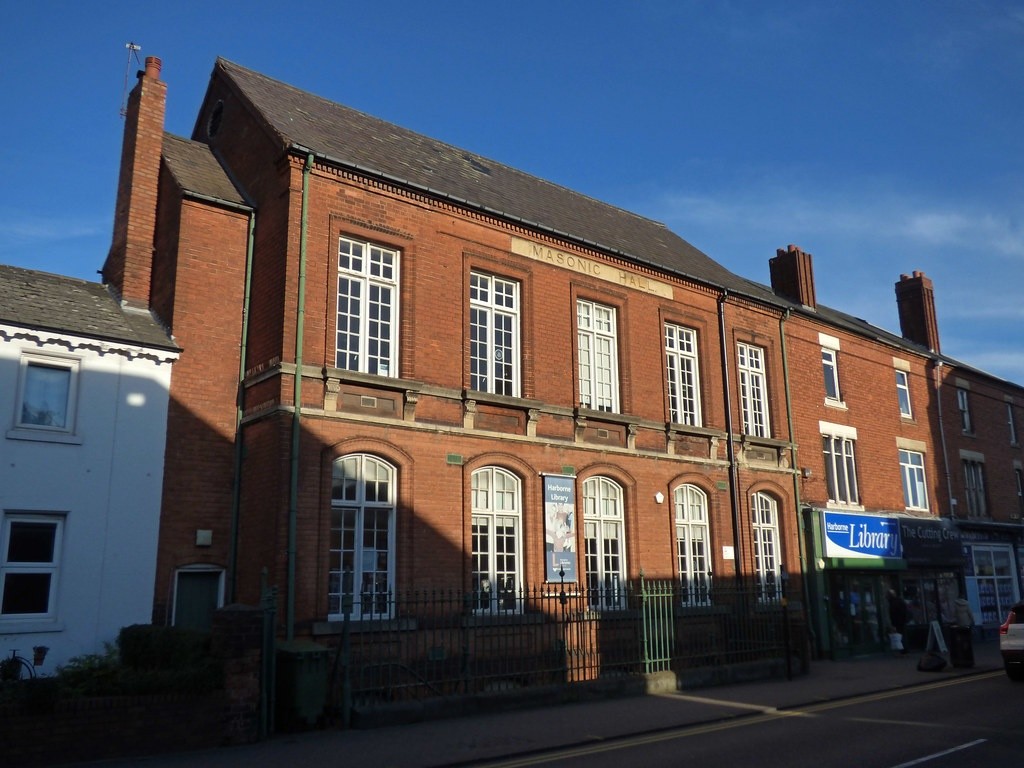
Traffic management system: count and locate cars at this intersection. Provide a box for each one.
[999,598,1024,683]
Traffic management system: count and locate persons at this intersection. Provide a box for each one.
[955,595,975,635]
[885,588,914,658]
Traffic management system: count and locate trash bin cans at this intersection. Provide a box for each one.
[975,626,983,642]
[275,640,331,722]
[950,625,973,667]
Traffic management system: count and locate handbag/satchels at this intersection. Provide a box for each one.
[889,630,904,650]
